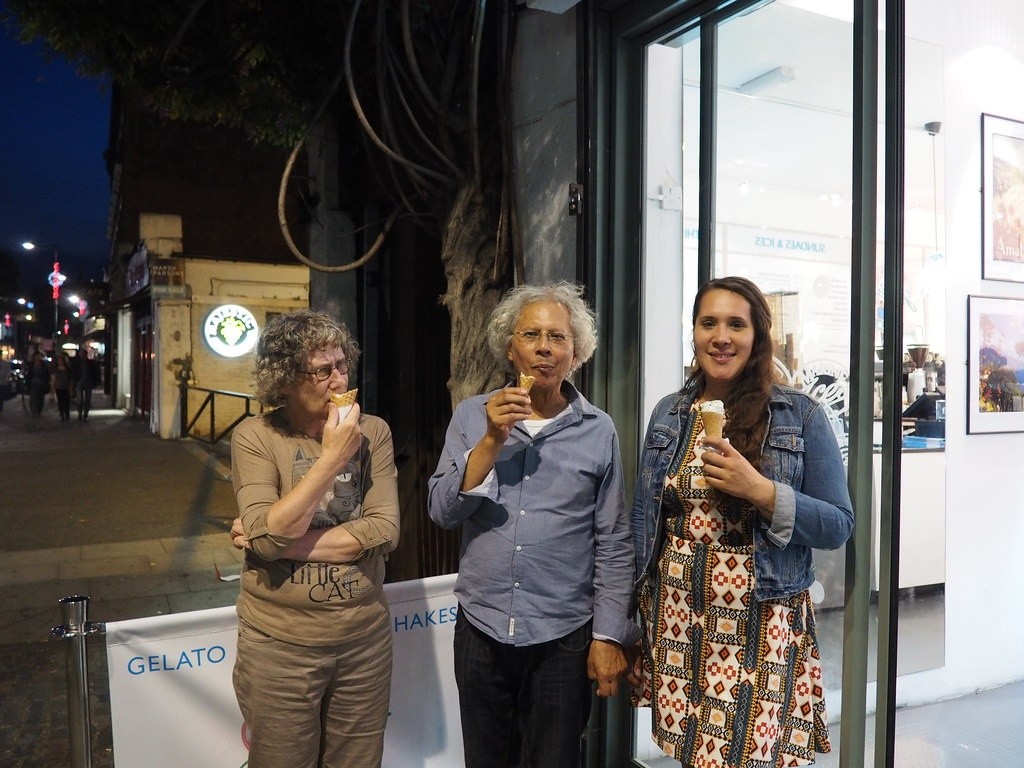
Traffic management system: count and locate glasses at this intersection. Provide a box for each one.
[296,357,353,381]
[510,330,574,347]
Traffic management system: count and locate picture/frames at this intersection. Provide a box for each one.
[981,112,1024,284]
[966,294,1024,435]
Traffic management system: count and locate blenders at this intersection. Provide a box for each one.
[907,344,934,405]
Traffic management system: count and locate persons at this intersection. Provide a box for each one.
[0,349,9,413]
[979,347,1020,412]
[73,349,99,422]
[51,357,74,423]
[622,276,855,768]
[231,307,401,768]
[26,351,51,418]
[427,283,644,768]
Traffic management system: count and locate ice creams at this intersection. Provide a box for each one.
[329,388,358,407]
[520,372,536,397]
[700,399,724,447]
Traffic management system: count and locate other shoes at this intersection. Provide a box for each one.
[84,418,88,422]
[61,418,65,423]
[66,415,70,421]
[78,415,82,421]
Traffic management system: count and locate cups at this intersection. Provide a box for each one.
[936,400,945,422]
